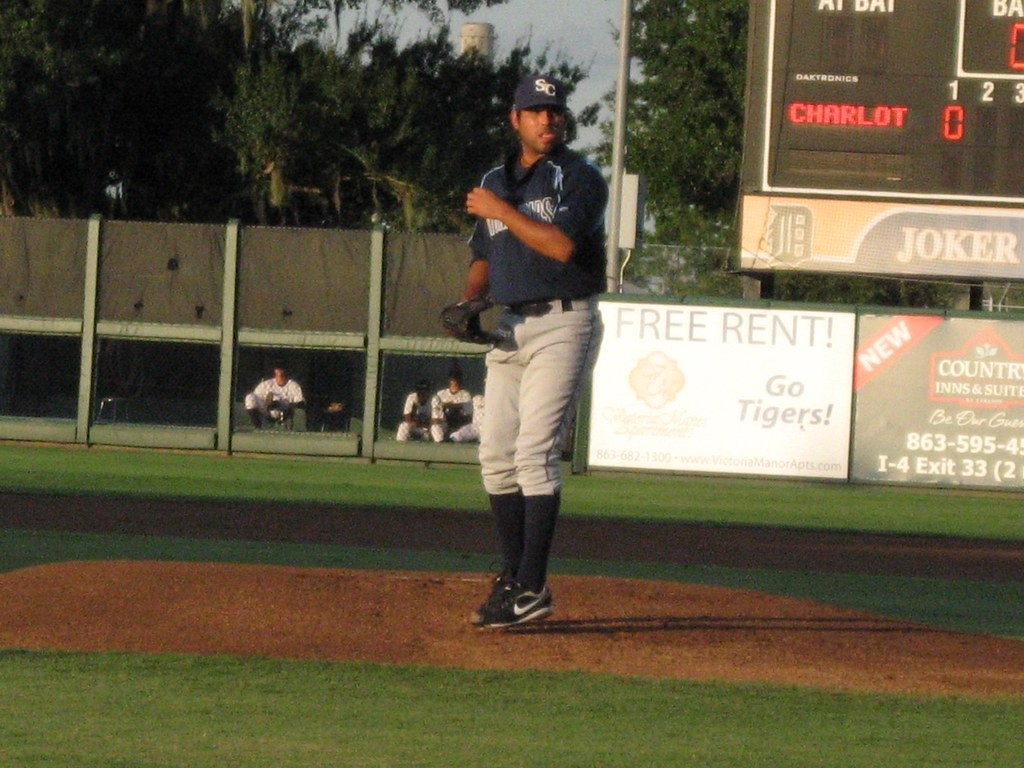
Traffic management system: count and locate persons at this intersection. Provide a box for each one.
[395,363,487,444]
[439,73,608,632]
[243,359,307,431]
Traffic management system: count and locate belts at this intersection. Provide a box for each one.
[506,297,572,317]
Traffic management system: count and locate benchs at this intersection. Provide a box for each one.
[125,395,308,433]
[345,410,403,440]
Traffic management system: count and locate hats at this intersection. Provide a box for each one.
[514,75,567,110]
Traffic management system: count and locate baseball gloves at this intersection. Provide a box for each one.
[441,296,505,346]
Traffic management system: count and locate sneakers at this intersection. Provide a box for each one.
[479,585,554,629]
[469,561,521,623]
